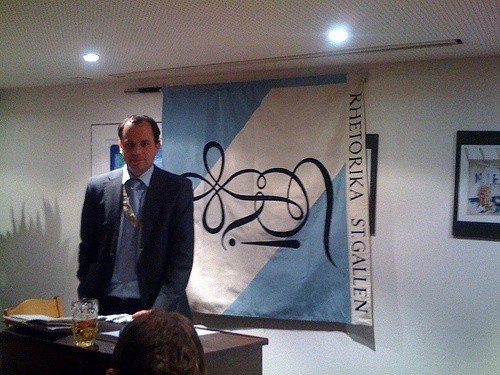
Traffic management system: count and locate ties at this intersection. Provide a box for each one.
[116,180,142,284]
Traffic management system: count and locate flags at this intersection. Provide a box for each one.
[162,67,373,327]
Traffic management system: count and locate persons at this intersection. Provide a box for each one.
[106,312,207,375]
[76,115,195,322]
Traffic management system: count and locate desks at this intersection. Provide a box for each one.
[0,323,268,375]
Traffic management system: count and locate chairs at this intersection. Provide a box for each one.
[3,295,64,328]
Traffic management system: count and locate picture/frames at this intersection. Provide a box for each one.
[365,133,378,236]
[451,130,500,242]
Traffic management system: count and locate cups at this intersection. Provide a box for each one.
[71,299,99,348]
